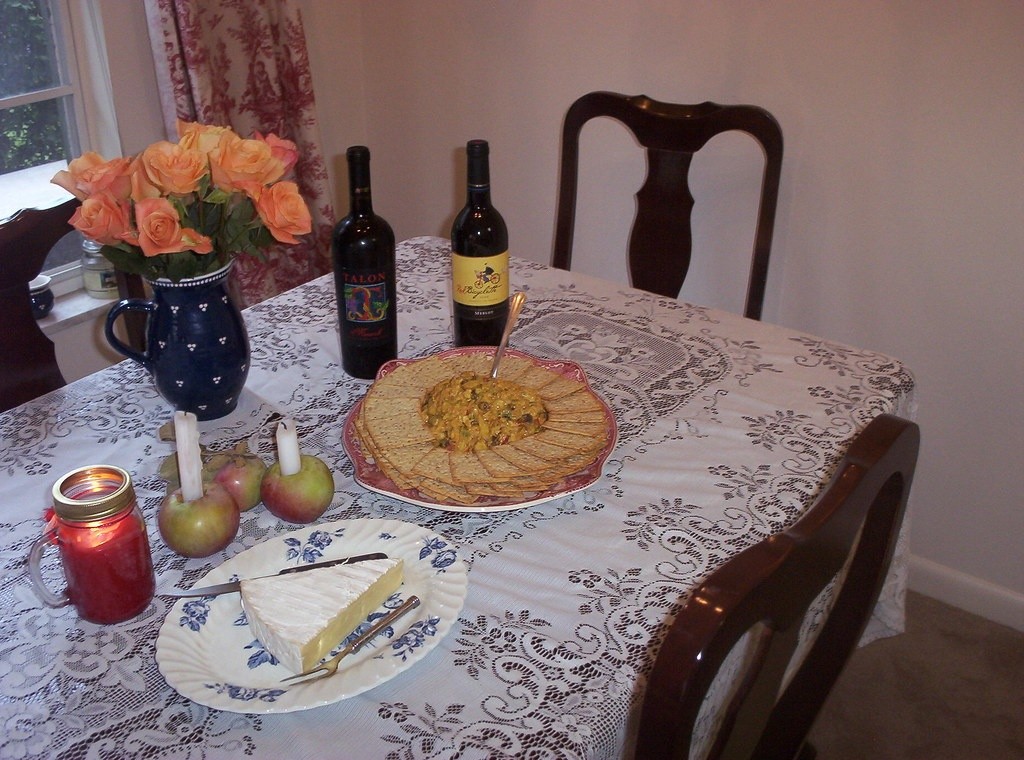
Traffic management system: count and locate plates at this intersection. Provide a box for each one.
[342,348,615,512]
[153,516,469,715]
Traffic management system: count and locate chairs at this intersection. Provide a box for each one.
[551,90,784,321]
[0,195,148,415]
[636,412,922,760]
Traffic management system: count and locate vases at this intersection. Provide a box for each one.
[105,256,251,421]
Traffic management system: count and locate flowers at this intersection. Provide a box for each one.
[51,120,312,285]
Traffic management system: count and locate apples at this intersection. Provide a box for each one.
[157,454,334,559]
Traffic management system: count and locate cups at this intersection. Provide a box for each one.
[28,274,55,321]
[28,464,155,625]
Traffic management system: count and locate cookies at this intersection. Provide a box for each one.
[352,355,610,505]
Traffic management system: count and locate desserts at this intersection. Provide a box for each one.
[240,558,404,675]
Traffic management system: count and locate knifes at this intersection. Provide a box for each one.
[160,552,389,606]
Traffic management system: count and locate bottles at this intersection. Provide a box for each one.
[82,238,120,300]
[450,140,509,353]
[329,146,399,381]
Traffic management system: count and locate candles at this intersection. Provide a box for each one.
[276,420,301,477]
[174,408,204,502]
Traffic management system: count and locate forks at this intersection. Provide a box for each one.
[280,594,422,686]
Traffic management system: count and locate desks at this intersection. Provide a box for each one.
[0,236,917,760]
